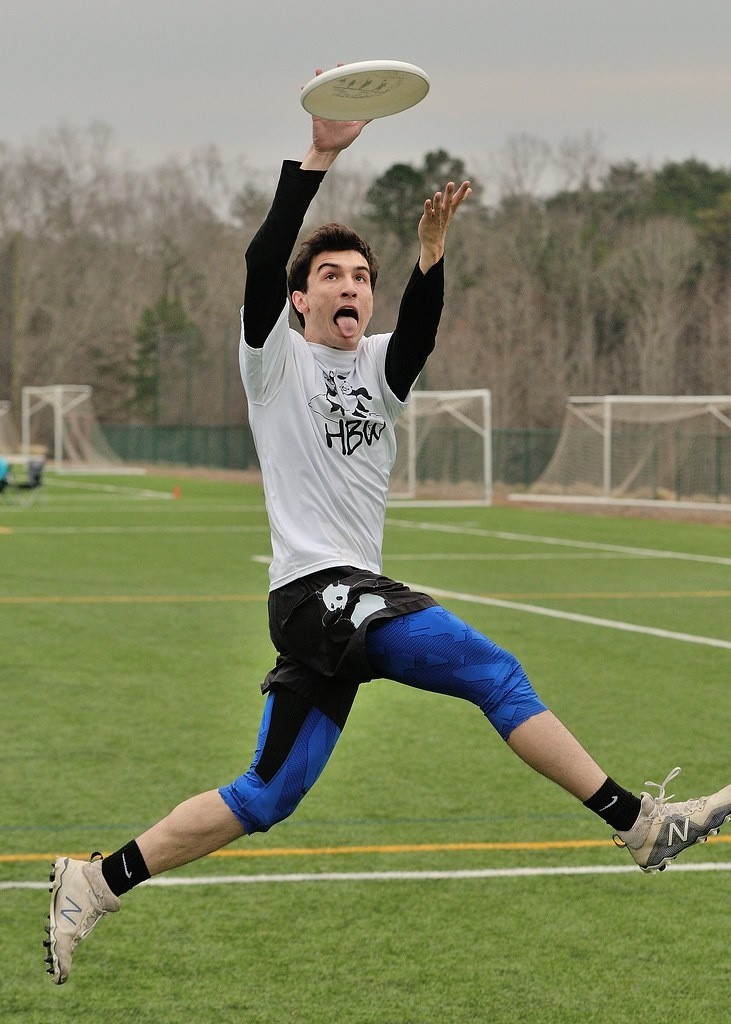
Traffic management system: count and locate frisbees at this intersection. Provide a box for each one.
[299,61,430,122]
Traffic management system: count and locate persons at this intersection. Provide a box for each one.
[45,64,731,982]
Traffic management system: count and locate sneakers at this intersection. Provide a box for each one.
[42,855,121,985]
[613,767,731,874]
